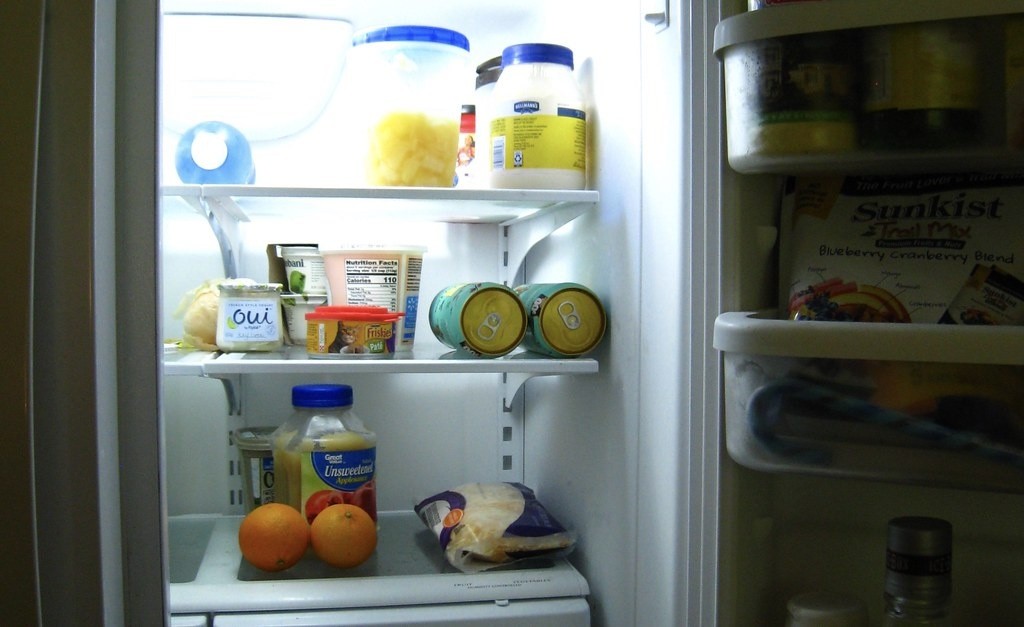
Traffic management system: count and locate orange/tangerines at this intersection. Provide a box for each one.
[239,503,311,572]
[311,504,376,570]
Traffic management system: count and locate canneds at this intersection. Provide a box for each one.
[215,282,284,352]
[429,281,527,357]
[511,281,606,358]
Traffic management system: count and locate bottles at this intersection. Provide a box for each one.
[884,517,954,627]
[272,384,380,558]
[177,122,256,184]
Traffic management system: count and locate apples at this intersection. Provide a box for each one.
[305,480,376,524]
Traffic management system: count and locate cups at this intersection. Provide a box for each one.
[344,26,470,188]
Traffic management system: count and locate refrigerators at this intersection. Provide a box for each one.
[94,0,1024,627]
